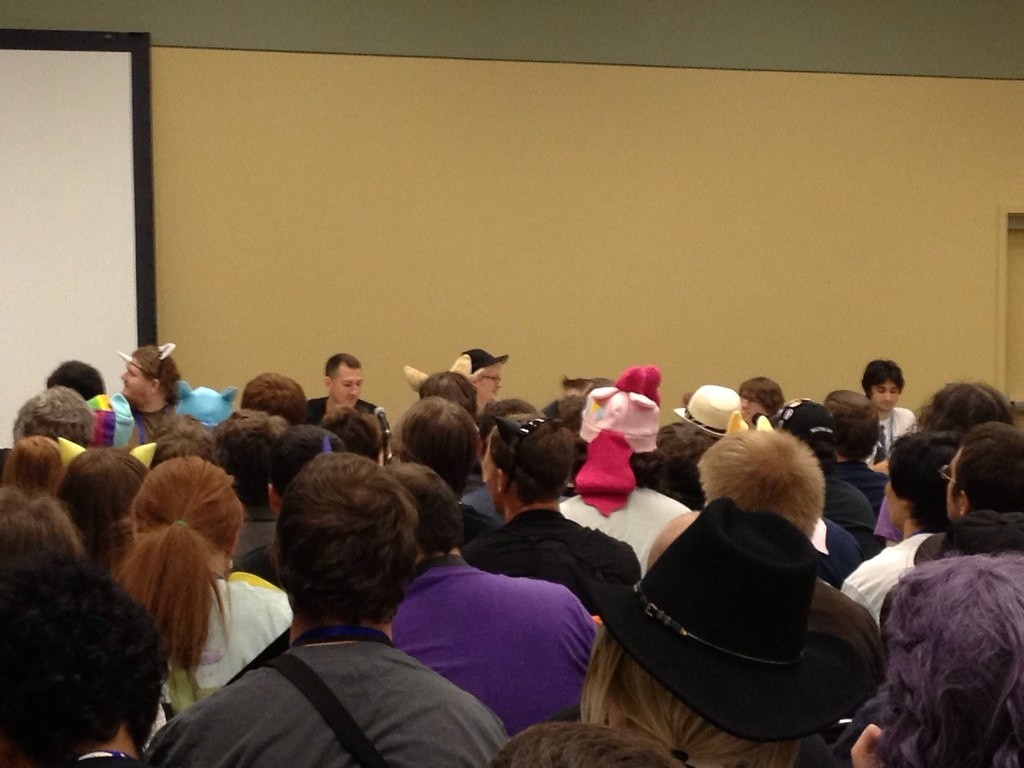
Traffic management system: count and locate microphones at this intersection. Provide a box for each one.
[375,407,390,435]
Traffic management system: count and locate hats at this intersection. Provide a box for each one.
[570,497,883,745]
[751,397,836,457]
[459,347,509,374]
[578,364,662,455]
[673,383,741,439]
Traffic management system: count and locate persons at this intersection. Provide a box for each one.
[0,347,1023,767]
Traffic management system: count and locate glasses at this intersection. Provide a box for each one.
[481,376,502,383]
[938,464,957,484]
